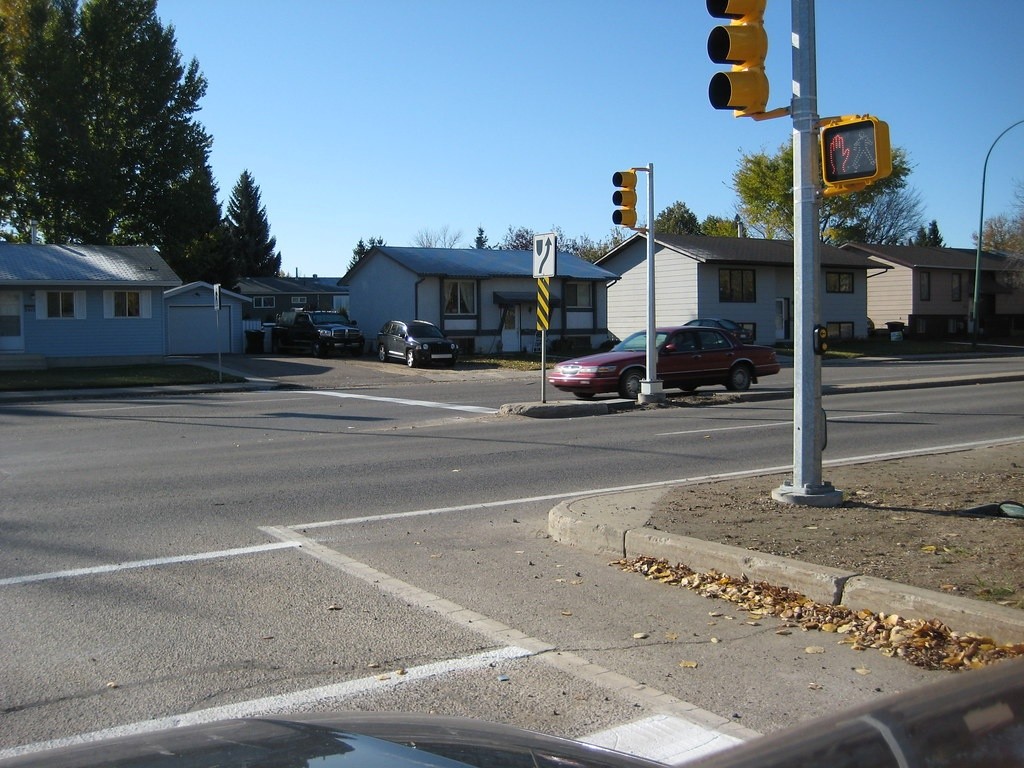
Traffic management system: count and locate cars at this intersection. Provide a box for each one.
[377,319,458,368]
[680,318,755,345]
[546,326,779,399]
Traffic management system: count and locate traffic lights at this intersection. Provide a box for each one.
[704,0,792,122]
[612,166,649,233]
[815,113,892,198]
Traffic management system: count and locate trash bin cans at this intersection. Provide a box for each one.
[244,329,265,354]
[885,322,905,343]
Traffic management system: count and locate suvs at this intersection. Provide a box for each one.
[272,310,365,358]
